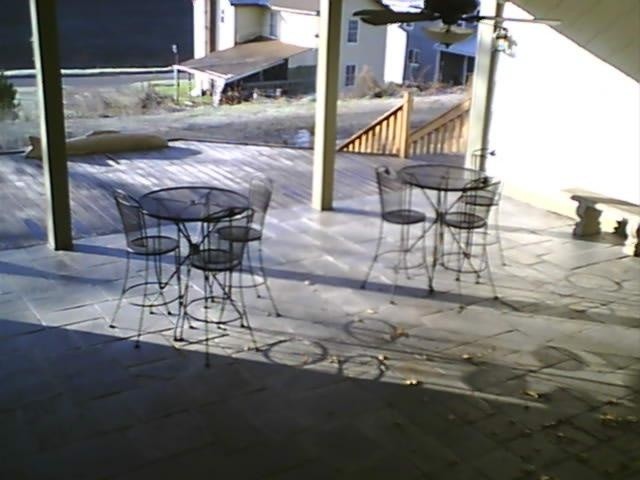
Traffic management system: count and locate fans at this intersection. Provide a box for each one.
[350,1,565,32]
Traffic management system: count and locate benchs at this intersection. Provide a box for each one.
[559,187,640,258]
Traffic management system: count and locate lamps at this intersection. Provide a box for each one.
[427,24,474,48]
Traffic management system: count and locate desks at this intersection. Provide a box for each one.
[395,161,493,286]
[138,186,256,334]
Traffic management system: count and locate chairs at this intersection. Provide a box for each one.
[360,166,434,305]
[174,203,266,369]
[423,175,500,316]
[105,185,196,350]
[444,146,511,285]
[204,175,287,330]
